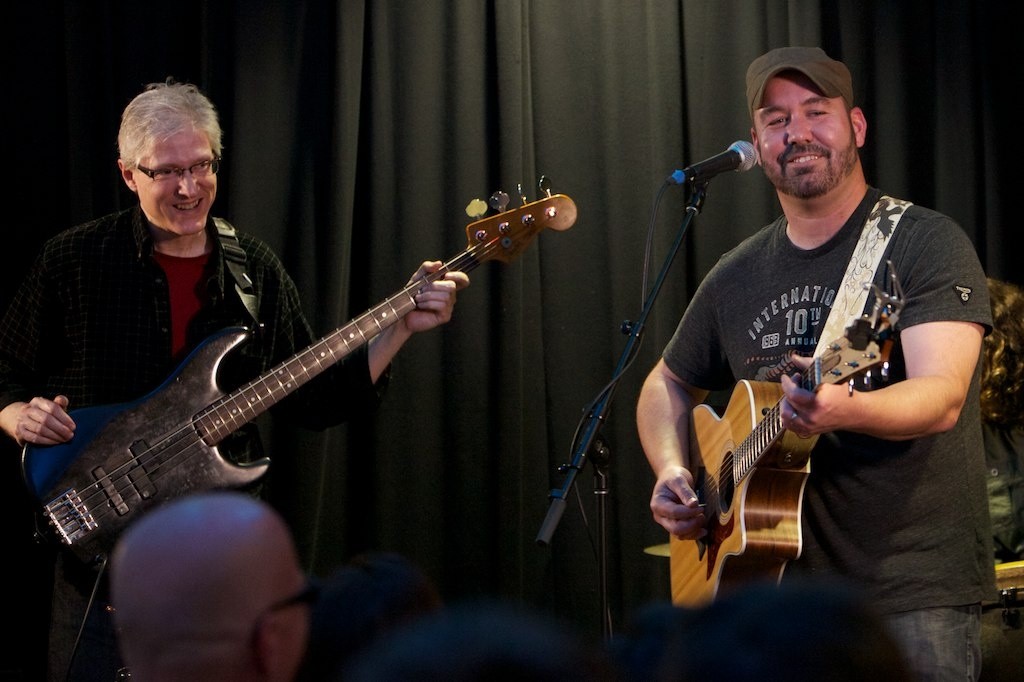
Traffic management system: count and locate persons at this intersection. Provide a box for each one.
[121,273,1024,682]
[636,47,1001,682]
[0,77,473,682]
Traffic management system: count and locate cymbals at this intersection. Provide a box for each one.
[643,543,670,558]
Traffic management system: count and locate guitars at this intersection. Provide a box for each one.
[18,173,582,575]
[669,313,894,608]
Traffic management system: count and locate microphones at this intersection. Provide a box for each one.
[665,140,759,185]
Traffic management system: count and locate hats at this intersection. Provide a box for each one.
[746,47,854,120]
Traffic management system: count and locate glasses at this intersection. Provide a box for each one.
[247,582,317,650]
[134,148,221,181]
